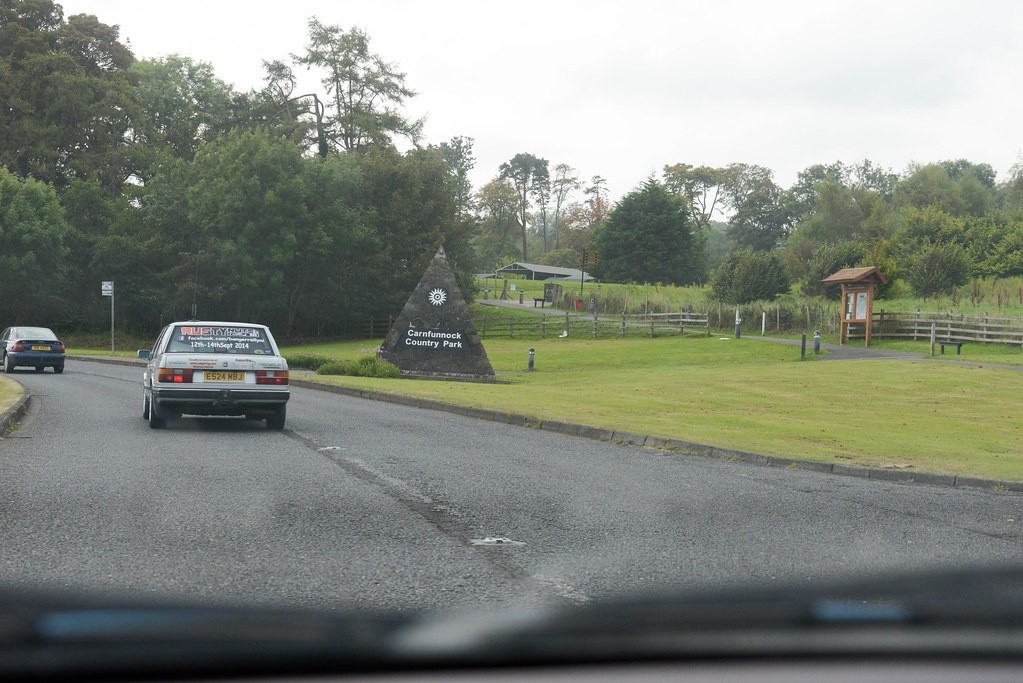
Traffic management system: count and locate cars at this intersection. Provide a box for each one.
[136,320,291,432]
[0,325,66,374]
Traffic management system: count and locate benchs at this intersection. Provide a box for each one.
[534,297,546,308]
[938,341,963,354]
